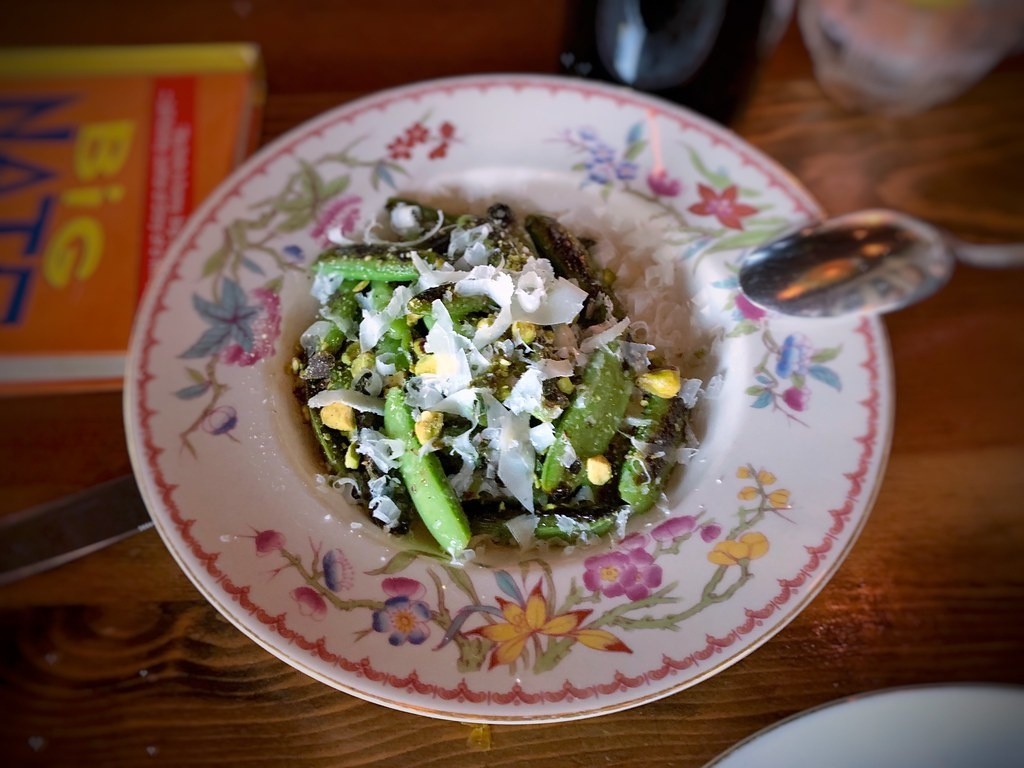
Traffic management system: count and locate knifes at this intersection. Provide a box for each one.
[1,473,159,591]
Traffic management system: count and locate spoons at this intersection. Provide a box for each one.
[736,204,1021,321]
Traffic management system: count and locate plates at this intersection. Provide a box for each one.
[120,72,898,729]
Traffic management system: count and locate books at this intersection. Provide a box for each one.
[0,44,268,389]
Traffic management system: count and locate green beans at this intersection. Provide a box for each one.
[289,198,686,557]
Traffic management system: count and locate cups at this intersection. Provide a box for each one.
[798,5,1023,120]
[552,1,792,129]
